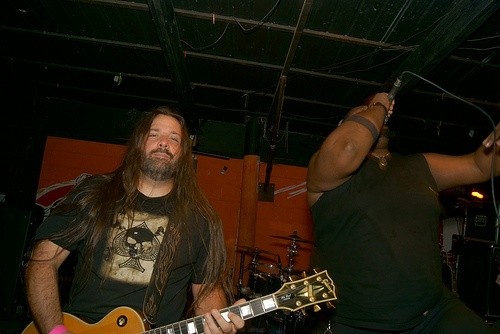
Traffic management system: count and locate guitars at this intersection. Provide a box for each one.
[23,264,336,333]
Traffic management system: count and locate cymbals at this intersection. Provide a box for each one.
[234,244,274,255]
[271,233,314,245]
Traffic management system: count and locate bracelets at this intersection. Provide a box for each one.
[370,102,389,124]
[48,324,70,334]
[342,115,379,143]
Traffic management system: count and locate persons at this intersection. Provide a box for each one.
[26,107,245,334]
[307,91,500,334]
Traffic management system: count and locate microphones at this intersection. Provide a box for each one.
[387,72,404,104]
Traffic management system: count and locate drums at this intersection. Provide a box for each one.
[248,263,282,291]
[236,268,317,334]
